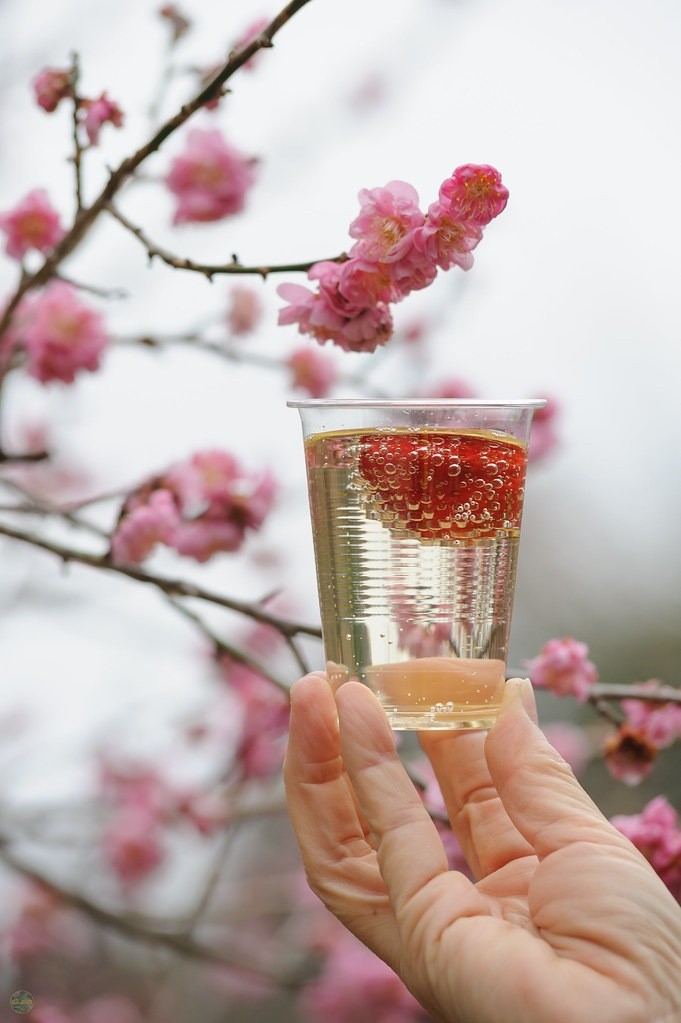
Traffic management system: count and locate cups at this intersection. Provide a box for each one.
[284,391,550,731]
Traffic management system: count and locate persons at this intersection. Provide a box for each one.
[283,667,681,1022]
